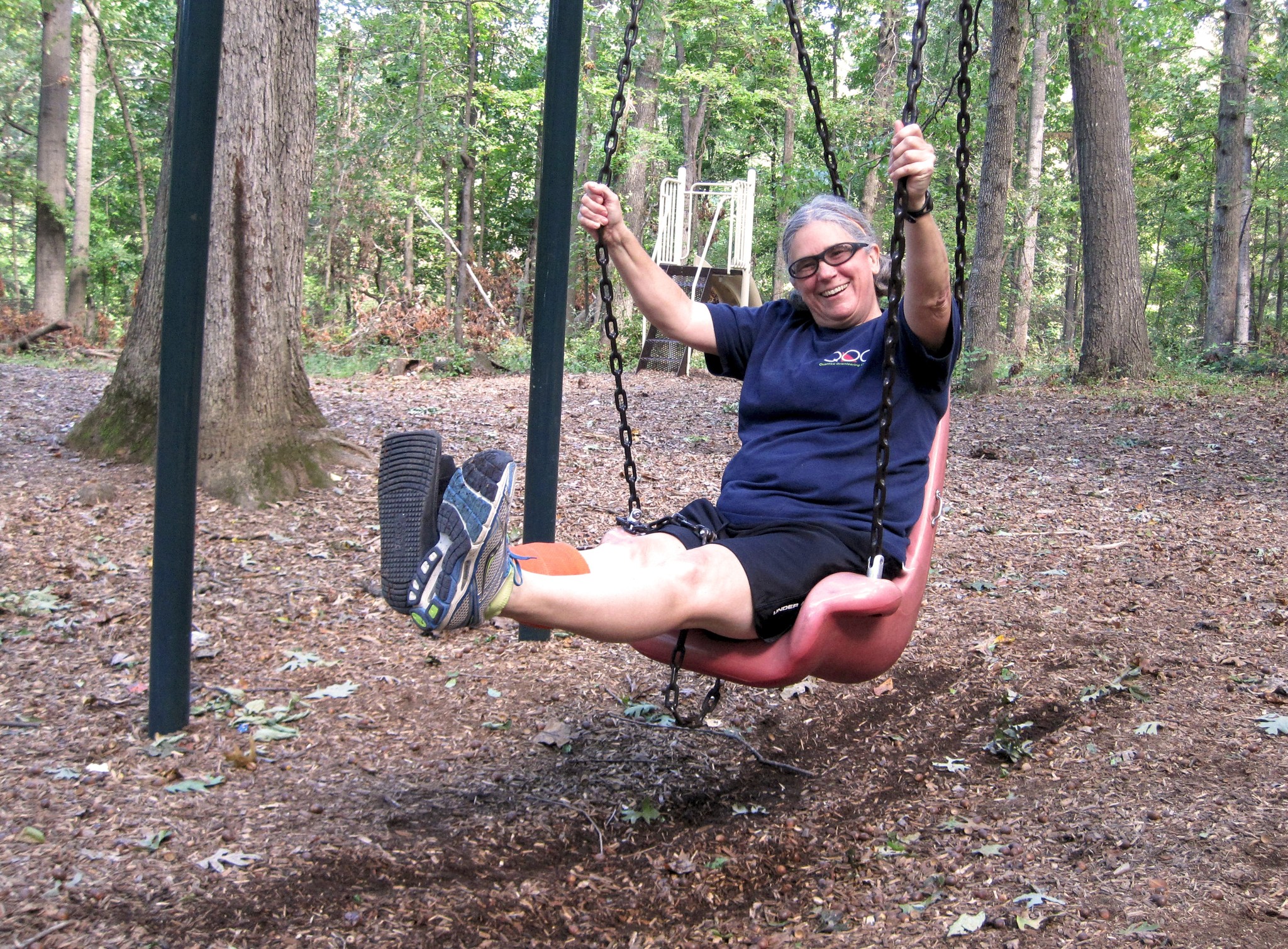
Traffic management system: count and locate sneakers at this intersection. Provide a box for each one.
[407,449,536,630]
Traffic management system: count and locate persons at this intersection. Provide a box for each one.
[376,119,957,641]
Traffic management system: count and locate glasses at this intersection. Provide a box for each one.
[788,241,868,279]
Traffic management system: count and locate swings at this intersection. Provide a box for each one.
[594,2,973,735]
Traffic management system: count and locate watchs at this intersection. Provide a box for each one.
[904,188,934,223]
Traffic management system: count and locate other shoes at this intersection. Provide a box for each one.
[377,431,455,618]
